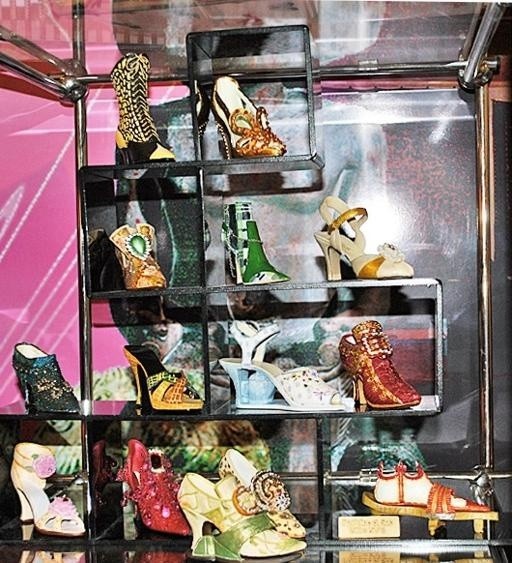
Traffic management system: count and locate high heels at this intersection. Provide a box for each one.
[178,471,307,559]
[212,75,288,159]
[10,442,85,537]
[339,321,421,408]
[220,202,292,285]
[314,195,414,281]
[217,449,307,539]
[220,321,353,411]
[123,345,204,411]
[109,222,167,288]
[89,438,118,517]
[115,440,190,536]
[12,342,80,413]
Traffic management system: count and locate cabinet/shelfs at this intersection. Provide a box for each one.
[0,25,509,556]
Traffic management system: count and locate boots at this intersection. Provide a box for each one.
[109,51,176,165]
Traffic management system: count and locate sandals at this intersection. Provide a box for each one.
[363,482,499,520]
